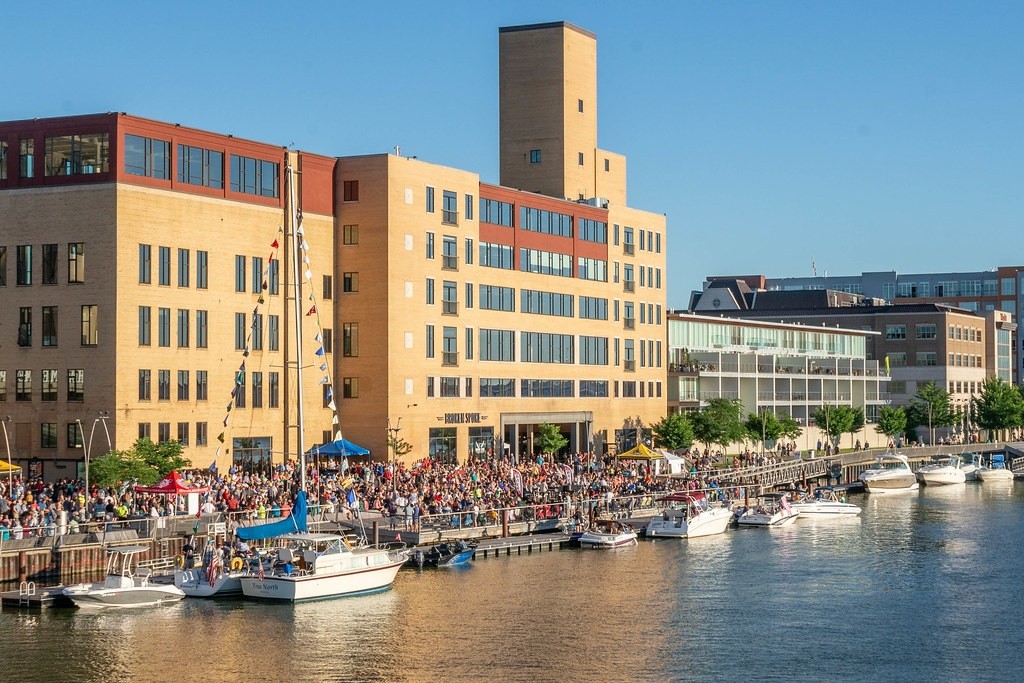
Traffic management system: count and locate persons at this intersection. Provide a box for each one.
[839,494,845,503]
[201,537,259,581]
[889,441,902,448]
[439,539,464,556]
[182,541,194,571]
[0,441,802,540]
[969,434,978,444]
[987,439,990,443]
[390,533,401,542]
[854,439,869,452]
[939,434,957,445]
[817,439,839,456]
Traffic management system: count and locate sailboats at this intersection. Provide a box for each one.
[173,165,419,606]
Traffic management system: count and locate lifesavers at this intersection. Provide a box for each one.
[173,554,184,569]
[230,556,243,571]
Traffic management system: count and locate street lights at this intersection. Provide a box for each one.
[823,400,831,455]
[760,404,769,457]
[964,403,971,445]
[583,411,593,473]
[385,417,402,496]
[927,401,934,446]
[75,418,100,511]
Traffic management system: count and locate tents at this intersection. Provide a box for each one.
[310,438,370,463]
[656,450,685,474]
[133,468,211,515]
[0,460,22,480]
[617,442,665,475]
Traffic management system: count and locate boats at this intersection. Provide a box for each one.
[737,489,801,529]
[62,544,187,610]
[404,536,479,570]
[912,439,1015,487]
[645,491,735,539]
[577,517,639,551]
[733,481,862,520]
[857,441,920,494]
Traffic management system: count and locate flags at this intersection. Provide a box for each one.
[192,240,279,534]
[207,548,220,588]
[298,224,358,508]
[259,560,264,580]
